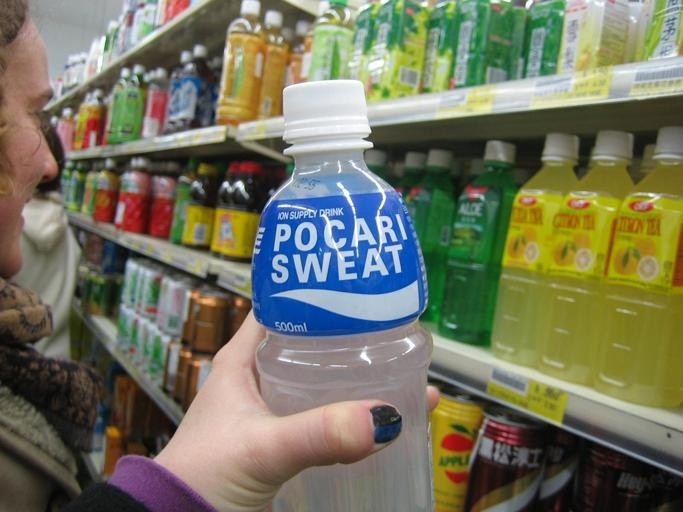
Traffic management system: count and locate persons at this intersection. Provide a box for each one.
[8,111,82,361]
[61,304,439,511]
[0,0,105,512]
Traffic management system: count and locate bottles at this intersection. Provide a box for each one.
[55,0,678,157]
[60,118,683,512]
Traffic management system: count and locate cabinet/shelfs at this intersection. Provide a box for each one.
[43,0,681,512]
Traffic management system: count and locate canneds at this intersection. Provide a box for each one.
[74,228,252,413]
[427,380,683,512]
